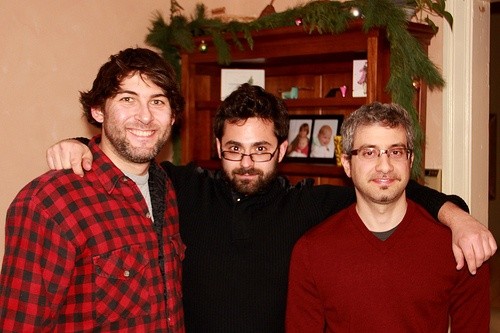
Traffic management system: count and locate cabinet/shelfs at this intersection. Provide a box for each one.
[173,16,440,187]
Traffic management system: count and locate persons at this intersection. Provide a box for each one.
[285,102,492,333]
[46,82,497,333]
[310,125,333,158]
[0,48,187,333]
[286,123,309,157]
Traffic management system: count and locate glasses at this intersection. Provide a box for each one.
[346,145,411,160]
[220,138,279,163]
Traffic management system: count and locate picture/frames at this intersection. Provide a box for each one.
[284,113,344,163]
